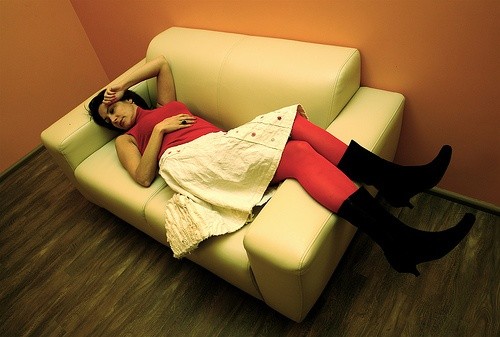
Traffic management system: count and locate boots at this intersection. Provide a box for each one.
[335,186,476,277]
[336,140,452,209]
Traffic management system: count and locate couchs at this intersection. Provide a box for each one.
[41,26,411,324]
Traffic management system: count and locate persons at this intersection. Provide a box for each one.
[85,55,476,277]
